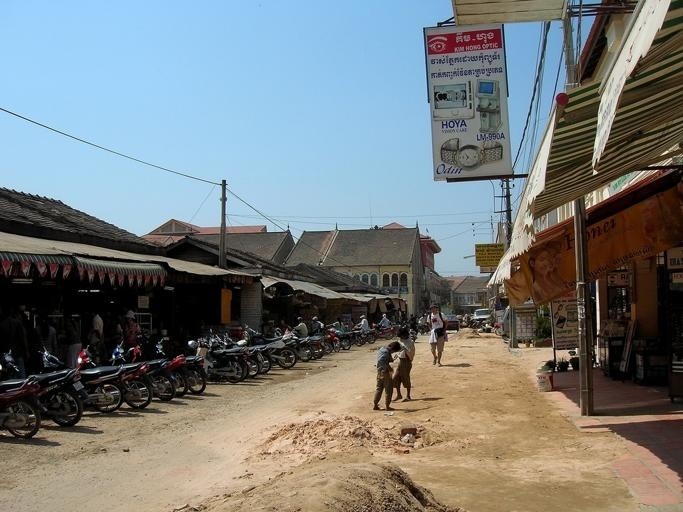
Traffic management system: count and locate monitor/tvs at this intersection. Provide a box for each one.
[475,79,499,109]
[431,80,474,120]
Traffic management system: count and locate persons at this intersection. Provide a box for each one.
[0,298,144,383]
[287,314,369,338]
[426,303,448,367]
[373,341,402,411]
[407,312,470,334]
[378,314,390,328]
[391,327,415,402]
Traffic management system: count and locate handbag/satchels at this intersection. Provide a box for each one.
[434,327,446,338]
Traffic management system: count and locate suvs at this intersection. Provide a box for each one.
[472,307,494,327]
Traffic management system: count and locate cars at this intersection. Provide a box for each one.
[442,313,460,332]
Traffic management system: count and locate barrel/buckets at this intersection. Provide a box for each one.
[536,371,553,393]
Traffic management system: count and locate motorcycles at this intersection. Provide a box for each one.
[190,314,429,387]
[0,323,206,440]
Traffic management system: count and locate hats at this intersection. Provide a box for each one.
[297,316,303,321]
[383,314,386,319]
[397,328,410,337]
[359,314,366,319]
[429,302,440,309]
